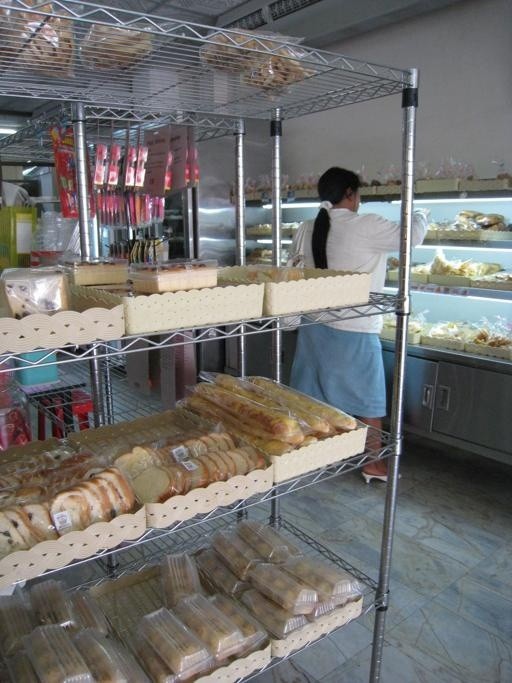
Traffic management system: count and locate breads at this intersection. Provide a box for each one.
[457,210,506,232]
[0,0,76,77]
[86,551,268,683]
[0,580,150,683]
[252,248,272,257]
[387,257,399,270]
[414,256,512,283]
[78,20,156,70]
[199,29,280,73]
[1,267,68,319]
[185,373,359,458]
[0,436,138,559]
[194,519,362,638]
[61,408,268,506]
[239,46,313,91]
[472,327,511,349]
[73,257,131,286]
[132,261,220,295]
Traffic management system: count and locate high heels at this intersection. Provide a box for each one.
[360,470,404,484]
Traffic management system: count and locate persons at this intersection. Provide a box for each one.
[278,167,430,484]
